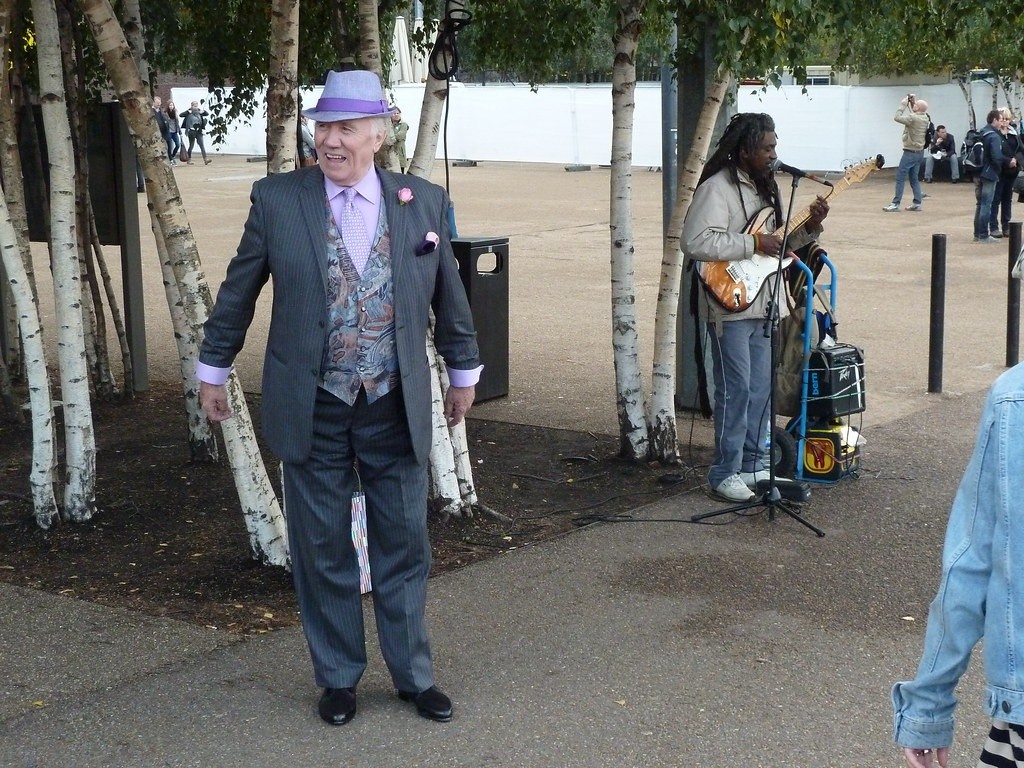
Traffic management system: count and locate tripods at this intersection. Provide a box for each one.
[691,175,827,538]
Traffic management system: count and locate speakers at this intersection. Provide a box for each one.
[804,344,866,418]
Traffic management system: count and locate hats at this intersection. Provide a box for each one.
[788,242,828,302]
[301,70,398,122]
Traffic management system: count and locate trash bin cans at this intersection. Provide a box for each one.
[447,235,511,405]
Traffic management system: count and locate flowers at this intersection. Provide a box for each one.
[398,187,414,206]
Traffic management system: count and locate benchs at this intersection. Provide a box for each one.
[917,156,973,181]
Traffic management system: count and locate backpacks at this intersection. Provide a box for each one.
[961,129,995,168]
[771,307,820,415]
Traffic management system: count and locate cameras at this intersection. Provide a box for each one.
[906,93,914,102]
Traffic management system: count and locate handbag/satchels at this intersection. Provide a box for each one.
[180,137,190,162]
[923,113,935,149]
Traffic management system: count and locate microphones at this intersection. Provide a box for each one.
[771,159,835,186]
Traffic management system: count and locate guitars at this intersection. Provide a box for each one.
[693,153,885,313]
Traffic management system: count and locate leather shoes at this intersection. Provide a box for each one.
[318,685,355,726]
[397,684,453,721]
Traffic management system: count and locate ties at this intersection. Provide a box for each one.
[341,188,373,279]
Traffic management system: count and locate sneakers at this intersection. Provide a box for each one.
[905,204,922,211]
[882,203,900,212]
[739,469,793,490]
[712,473,756,503]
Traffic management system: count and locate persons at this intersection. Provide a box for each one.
[152,94,170,138]
[163,101,183,167]
[189,67,485,722]
[679,109,833,502]
[391,106,410,174]
[180,100,212,166]
[922,125,960,184]
[297,112,319,167]
[881,95,930,212]
[891,241,1024,768]
[960,106,1024,239]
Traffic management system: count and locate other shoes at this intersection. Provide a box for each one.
[187,161,194,165]
[973,235,999,244]
[952,179,957,183]
[1002,229,1009,237]
[170,159,177,166]
[927,178,932,182]
[205,159,212,165]
[990,230,1003,237]
